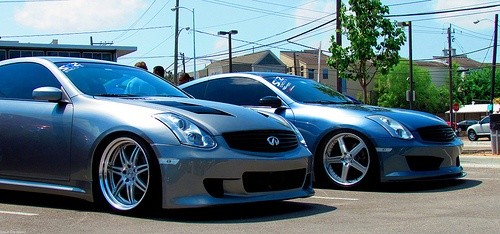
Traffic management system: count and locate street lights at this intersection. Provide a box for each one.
[398,21,414,110]
[217,29,238,73]
[173,1,191,83]
[171,6,198,79]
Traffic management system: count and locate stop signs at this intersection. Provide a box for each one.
[453,103,461,113]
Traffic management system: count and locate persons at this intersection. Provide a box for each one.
[493,97,500,114]
[179,73,194,85]
[124,62,153,95]
[153,66,164,78]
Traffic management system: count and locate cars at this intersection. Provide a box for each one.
[0,56,316,215]
[466,115,491,141]
[177,71,466,192]
[457,120,476,131]
